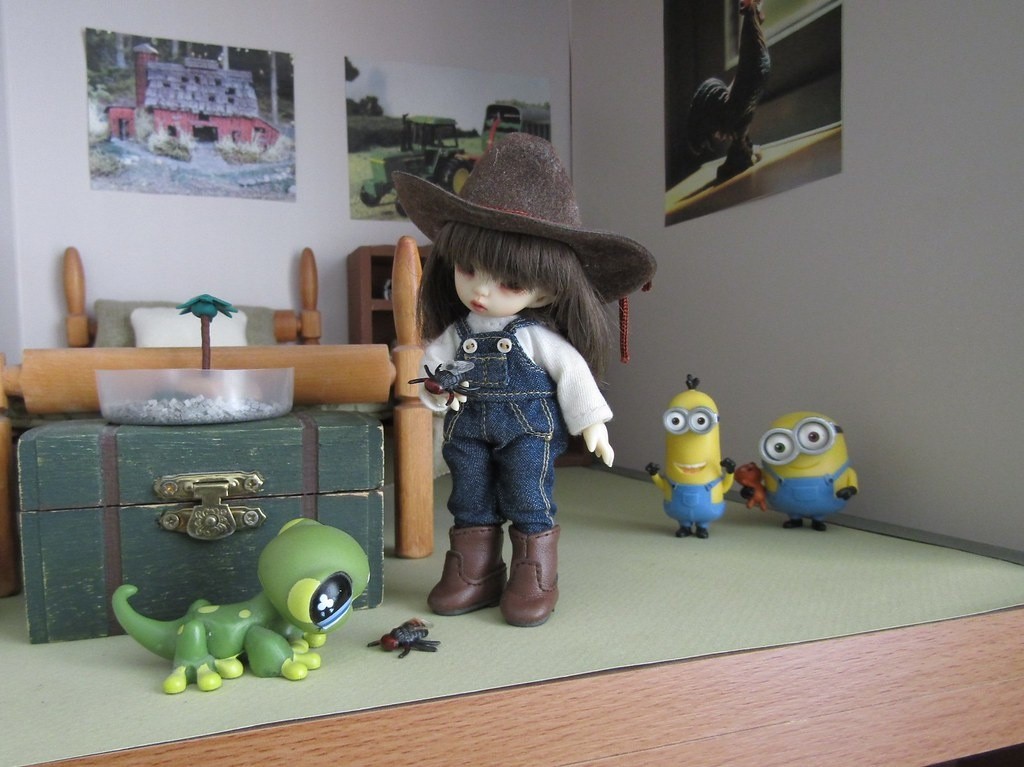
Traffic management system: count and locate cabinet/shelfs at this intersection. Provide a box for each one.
[345,244,593,467]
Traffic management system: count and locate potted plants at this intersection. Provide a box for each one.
[93,291,294,429]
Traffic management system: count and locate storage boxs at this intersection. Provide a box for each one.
[15,408,388,645]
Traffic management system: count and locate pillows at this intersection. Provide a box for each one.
[91,298,278,348]
[128,306,249,348]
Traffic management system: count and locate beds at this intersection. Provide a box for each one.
[0,235,439,599]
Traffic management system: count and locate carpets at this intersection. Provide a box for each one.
[0,462,1024,767]
